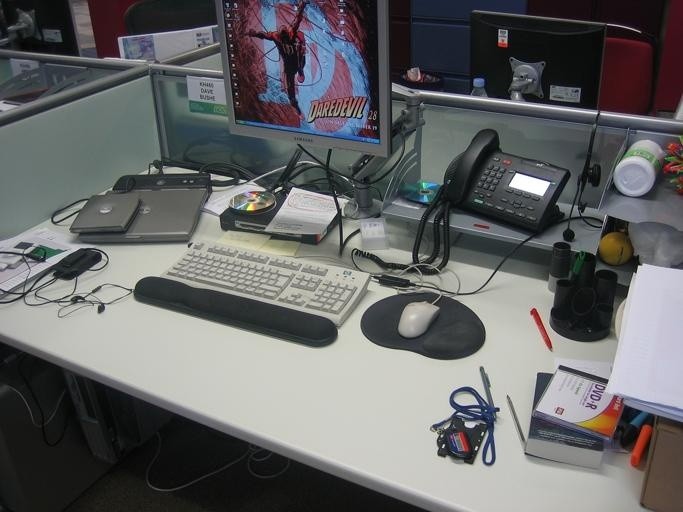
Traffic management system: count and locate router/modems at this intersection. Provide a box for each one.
[220,192,342,245]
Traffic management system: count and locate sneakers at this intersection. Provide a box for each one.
[296,114,305,122]
[297,71,305,83]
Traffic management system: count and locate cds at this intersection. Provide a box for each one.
[227,190,276,211]
[400,179,442,204]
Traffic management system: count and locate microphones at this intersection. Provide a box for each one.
[562,175,580,241]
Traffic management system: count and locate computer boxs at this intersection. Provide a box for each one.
[0,352,134,512]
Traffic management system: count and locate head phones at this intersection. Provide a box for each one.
[580,110,601,188]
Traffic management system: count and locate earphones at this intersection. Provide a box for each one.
[70,296,85,302]
[97,304,104,313]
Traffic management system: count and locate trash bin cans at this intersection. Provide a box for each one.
[400,71,443,90]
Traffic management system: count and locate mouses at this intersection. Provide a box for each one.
[397,300,440,339]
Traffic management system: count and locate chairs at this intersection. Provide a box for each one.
[122,0,205,38]
[600,20,659,115]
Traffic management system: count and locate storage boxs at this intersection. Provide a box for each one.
[641,417,682,512]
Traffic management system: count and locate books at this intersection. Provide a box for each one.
[0,226,79,302]
[602,263,683,426]
[529,364,625,443]
[521,370,607,472]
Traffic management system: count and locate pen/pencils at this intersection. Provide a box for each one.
[567,251,586,283]
[507,395,525,442]
[530,307,553,352]
[622,406,654,466]
[480,366,497,421]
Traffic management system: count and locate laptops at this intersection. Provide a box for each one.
[77,189,208,243]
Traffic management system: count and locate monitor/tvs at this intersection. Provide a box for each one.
[215,0,392,159]
[0,0,81,59]
[469,10,606,110]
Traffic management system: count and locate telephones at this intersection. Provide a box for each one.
[444,129,571,233]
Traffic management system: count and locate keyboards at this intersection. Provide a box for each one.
[159,239,371,328]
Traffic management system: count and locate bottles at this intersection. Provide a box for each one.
[615,138,665,198]
[470,77,487,97]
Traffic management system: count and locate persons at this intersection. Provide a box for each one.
[241,0,310,118]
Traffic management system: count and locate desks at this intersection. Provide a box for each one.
[0,153,648,512]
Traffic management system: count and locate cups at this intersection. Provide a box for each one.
[546,240,619,342]
